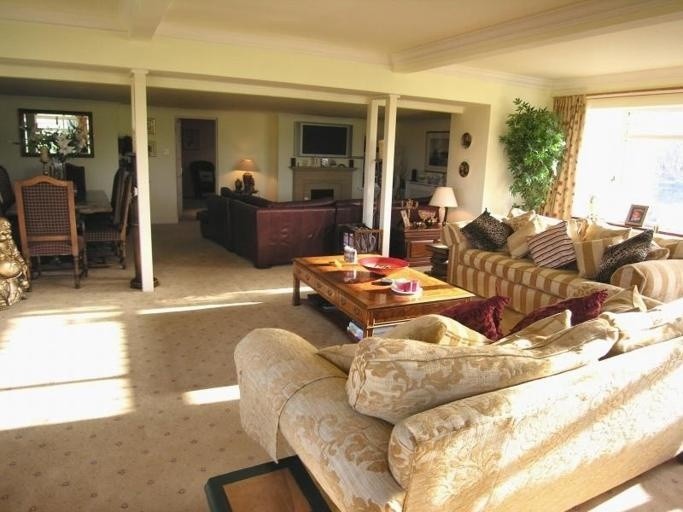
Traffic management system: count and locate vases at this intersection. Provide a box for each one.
[147,117,157,158]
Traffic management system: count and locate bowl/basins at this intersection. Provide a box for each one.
[358,257,409,276]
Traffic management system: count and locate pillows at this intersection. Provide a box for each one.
[461,209,670,283]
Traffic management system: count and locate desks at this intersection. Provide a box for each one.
[398,223,446,267]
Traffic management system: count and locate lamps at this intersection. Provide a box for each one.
[428,186,459,225]
[235,159,259,196]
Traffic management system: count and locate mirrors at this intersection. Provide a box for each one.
[18,108,94,158]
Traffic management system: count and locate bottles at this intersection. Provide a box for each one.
[343,268,357,282]
[235,179,241,192]
[344,246,357,263]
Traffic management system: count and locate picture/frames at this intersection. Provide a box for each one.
[424,131,450,174]
[625,204,649,227]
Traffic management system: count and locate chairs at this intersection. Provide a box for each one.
[0,163,133,289]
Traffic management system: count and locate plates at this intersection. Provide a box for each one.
[460,132,472,149]
[459,161,469,177]
[390,279,423,295]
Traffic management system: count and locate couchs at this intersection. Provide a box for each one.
[441,208,683,315]
[207,187,439,269]
[233,327,683,512]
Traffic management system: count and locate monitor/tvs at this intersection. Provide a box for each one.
[300,123,350,158]
